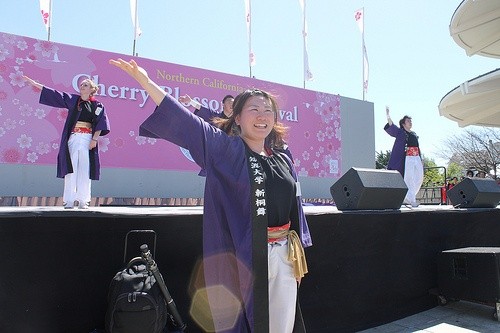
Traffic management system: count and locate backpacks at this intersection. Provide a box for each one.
[105,257,167,333]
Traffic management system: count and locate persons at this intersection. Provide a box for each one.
[383,105,424,207]
[109,58,312,333]
[178,94,235,135]
[19,75,110,208]
[442,170,500,205]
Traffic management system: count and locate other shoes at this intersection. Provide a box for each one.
[64,201,74,209]
[409,201,418,207]
[78,201,89,209]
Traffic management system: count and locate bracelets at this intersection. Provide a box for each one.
[92,138,98,142]
[190,100,198,107]
